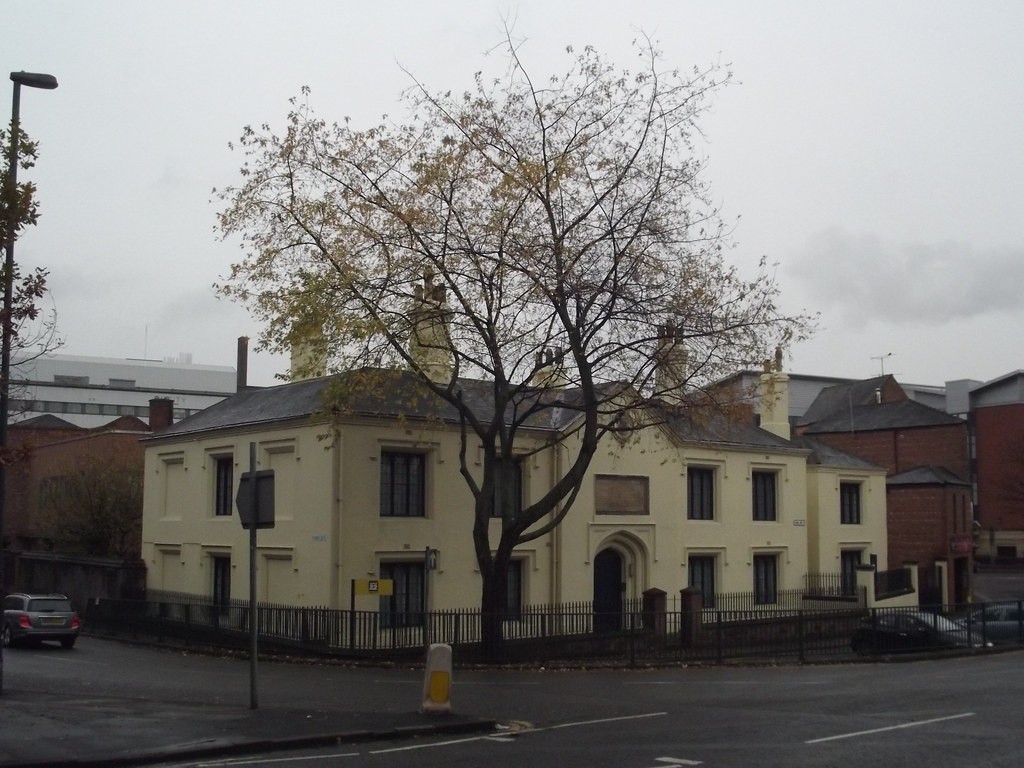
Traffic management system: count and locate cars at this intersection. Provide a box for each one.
[948,602,1022,645]
[851,610,995,657]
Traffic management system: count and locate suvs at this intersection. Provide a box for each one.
[2,591,82,650]
